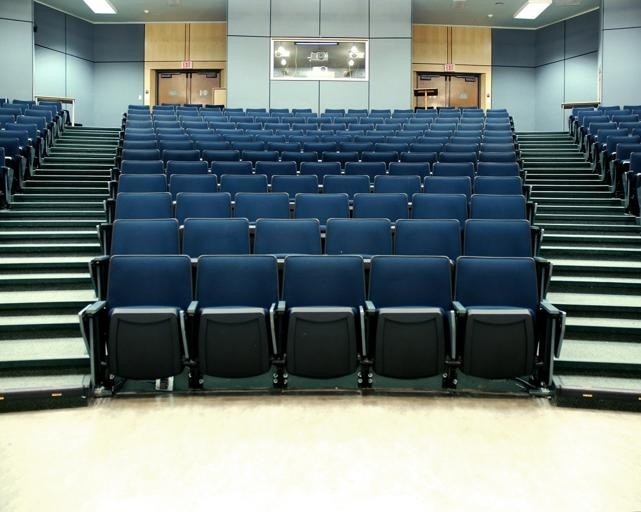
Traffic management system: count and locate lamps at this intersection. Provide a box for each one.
[84,0,121,16]
[513,0,554,20]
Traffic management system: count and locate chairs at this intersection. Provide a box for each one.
[77,100,567,402]
[567,101,641,216]
[0,94,71,210]
